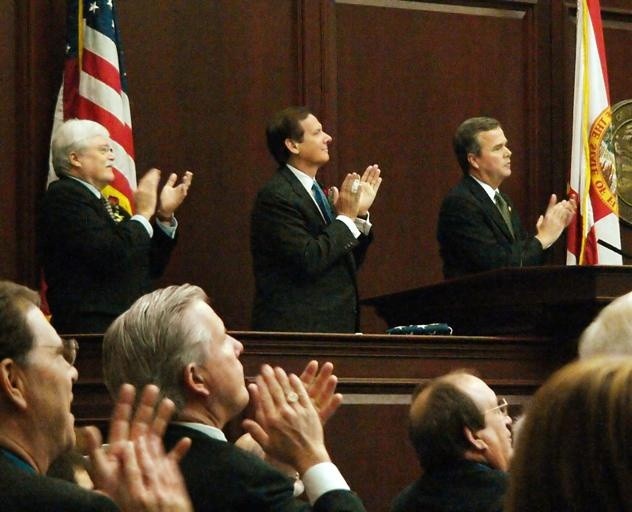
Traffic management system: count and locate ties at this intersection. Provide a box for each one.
[493,190,515,241]
[310,182,333,226]
[98,188,114,219]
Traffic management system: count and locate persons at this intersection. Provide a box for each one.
[436,116,578,281]
[387,373,513,512]
[0,280,193,512]
[103,282,366,512]
[248,104,383,334]
[504,357,632,512]
[34,118,194,334]
[576,288,632,361]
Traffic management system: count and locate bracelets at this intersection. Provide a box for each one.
[355,214,368,221]
[158,212,174,223]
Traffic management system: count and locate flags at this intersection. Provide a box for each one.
[566,0,624,267]
[39,0,138,322]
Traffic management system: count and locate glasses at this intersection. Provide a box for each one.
[482,395,510,419]
[37,334,79,365]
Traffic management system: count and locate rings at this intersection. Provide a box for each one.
[286,391,299,403]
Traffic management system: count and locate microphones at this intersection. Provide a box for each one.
[594,240,631,261]
